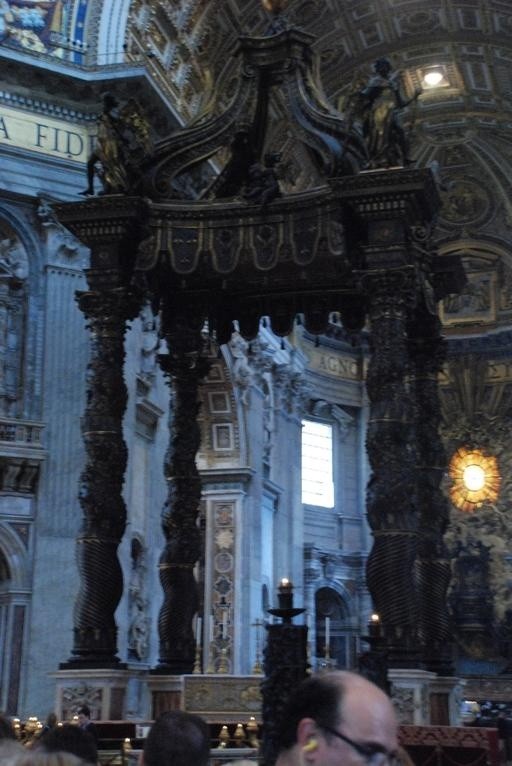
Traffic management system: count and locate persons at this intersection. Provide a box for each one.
[259,671,399,766]
[77,91,135,196]
[0,707,100,766]
[137,710,209,766]
[350,57,423,169]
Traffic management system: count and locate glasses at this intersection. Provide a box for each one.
[314,718,398,766]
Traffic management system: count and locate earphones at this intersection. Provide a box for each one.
[301,736,319,754]
